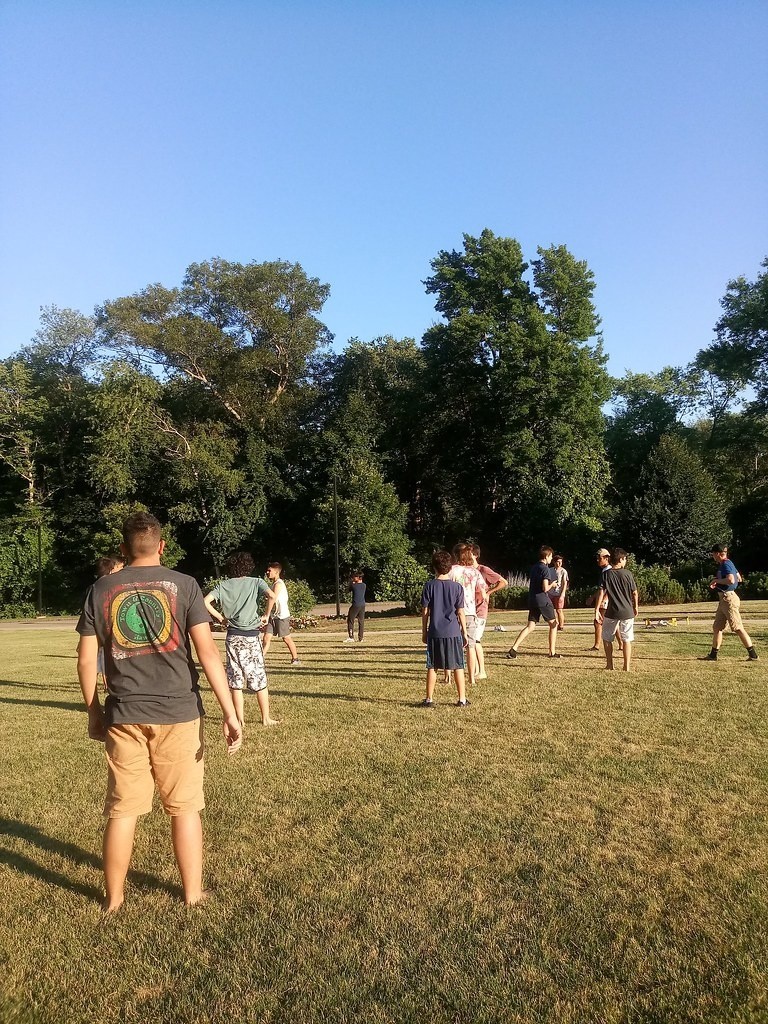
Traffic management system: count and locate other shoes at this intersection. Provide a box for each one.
[549,652,565,658]
[418,699,434,708]
[585,646,599,652]
[697,654,717,662]
[557,625,563,632]
[458,699,471,707]
[506,648,517,659]
[745,656,759,661]
[291,658,301,666]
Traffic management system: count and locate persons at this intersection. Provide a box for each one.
[442,544,509,684]
[261,562,301,664]
[697,544,758,661]
[93,553,126,692]
[76,511,242,912]
[595,548,638,671]
[506,546,563,658]
[204,552,283,727]
[585,548,624,651]
[343,571,366,643]
[547,555,569,630]
[421,550,471,706]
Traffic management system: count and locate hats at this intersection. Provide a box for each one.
[710,543,728,553]
[597,548,611,557]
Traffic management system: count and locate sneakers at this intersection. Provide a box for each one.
[343,637,355,643]
